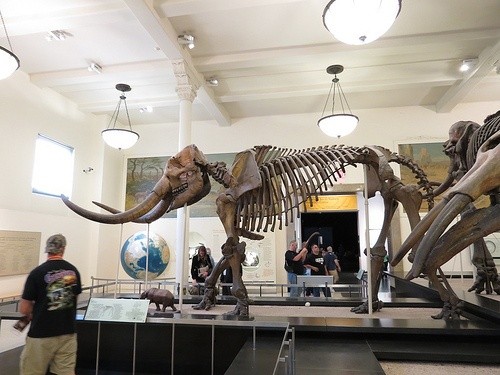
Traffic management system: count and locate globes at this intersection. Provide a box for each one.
[119,232,171,281]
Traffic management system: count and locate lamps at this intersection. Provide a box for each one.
[317,64,359,139]
[0,10,20,80]
[101,84,140,151]
[322,0,402,46]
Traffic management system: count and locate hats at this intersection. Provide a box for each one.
[44,234,66,254]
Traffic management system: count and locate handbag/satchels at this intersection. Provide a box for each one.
[198,266,210,278]
[220,274,226,283]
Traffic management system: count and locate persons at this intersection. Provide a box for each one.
[220,264,242,296]
[18,234,82,375]
[190,246,213,285]
[285,240,341,297]
